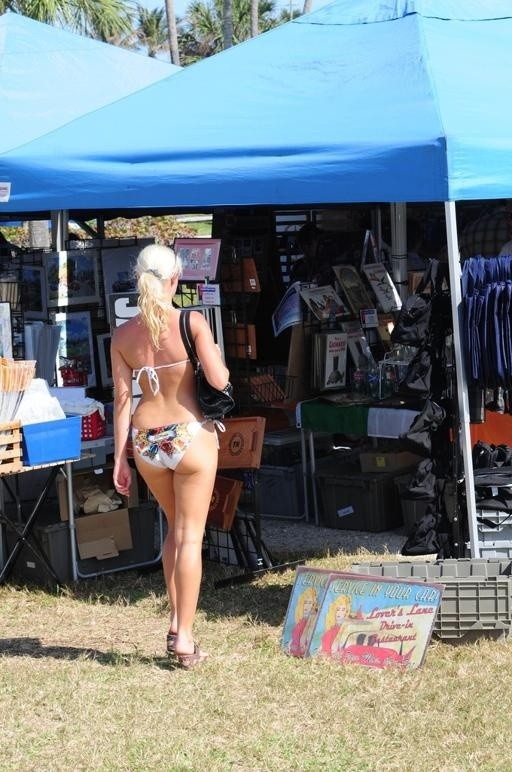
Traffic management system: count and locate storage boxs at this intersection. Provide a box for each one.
[243,451,424,533]
[54,461,141,561]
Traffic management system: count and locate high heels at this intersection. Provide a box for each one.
[166,634,177,651]
[178,642,209,671]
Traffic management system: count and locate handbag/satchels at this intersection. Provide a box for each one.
[461,255,512,391]
[196,364,235,419]
[389,256,457,556]
[473,440,512,528]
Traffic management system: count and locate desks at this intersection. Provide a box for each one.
[0,451,97,587]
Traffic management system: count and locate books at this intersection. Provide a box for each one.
[269,260,403,391]
[1,264,102,466]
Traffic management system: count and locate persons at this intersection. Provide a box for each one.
[317,593,352,656]
[287,587,319,658]
[110,243,238,671]
